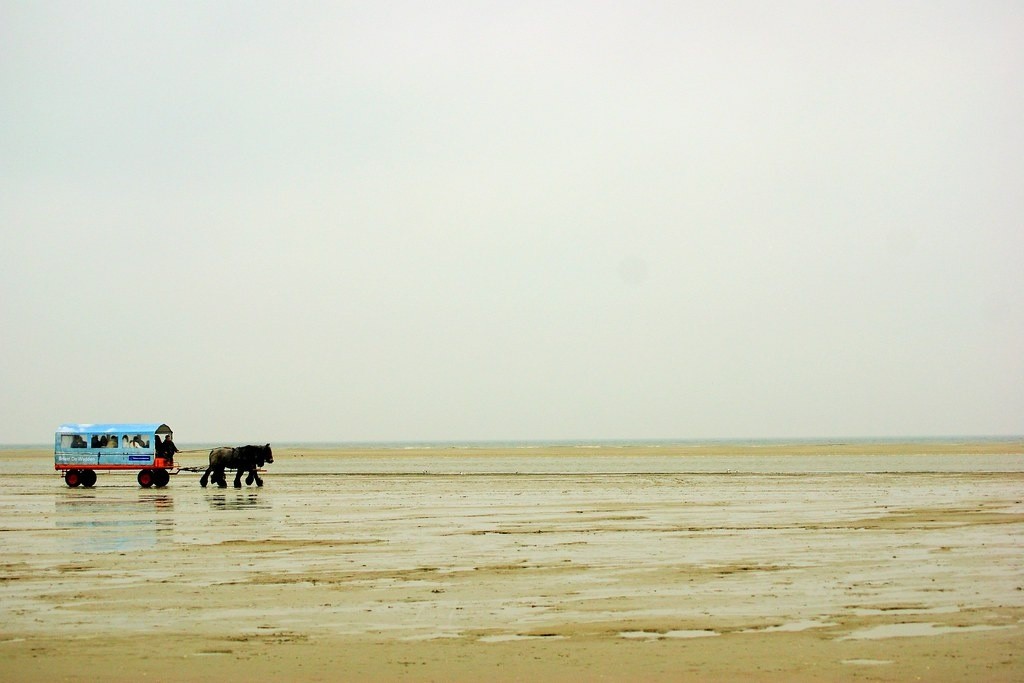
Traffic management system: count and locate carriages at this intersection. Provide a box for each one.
[55,424,273,488]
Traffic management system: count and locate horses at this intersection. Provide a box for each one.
[200,444,274,489]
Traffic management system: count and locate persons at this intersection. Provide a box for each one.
[70,435,180,466]
